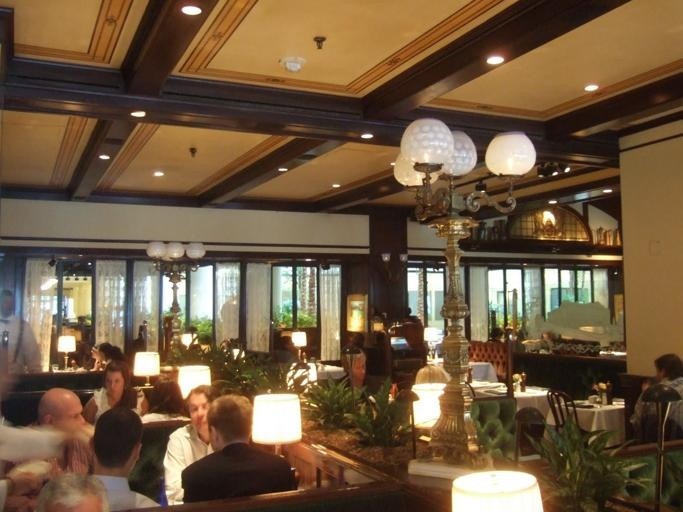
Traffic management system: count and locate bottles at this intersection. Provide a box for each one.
[521,372,527,392]
[606,381,613,404]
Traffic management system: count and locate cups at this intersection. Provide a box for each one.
[52,364,59,373]
[310,356,316,363]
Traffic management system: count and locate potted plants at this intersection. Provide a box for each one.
[349,381,419,465]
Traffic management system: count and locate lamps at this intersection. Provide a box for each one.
[282,56,305,72]
[177,364,211,401]
[451,471,543,512]
[397,252,408,286]
[537,157,572,178]
[56,334,77,370]
[382,252,394,285]
[393,116,537,481]
[291,331,308,361]
[133,351,161,386]
[146,241,206,364]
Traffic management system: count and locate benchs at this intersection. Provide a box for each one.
[5,370,409,512]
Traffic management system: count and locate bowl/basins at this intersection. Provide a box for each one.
[588,395,598,404]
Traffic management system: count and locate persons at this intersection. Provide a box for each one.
[480,325,566,356]
[1,287,41,377]
[340,331,384,374]
[632,353,683,431]
[0,335,299,512]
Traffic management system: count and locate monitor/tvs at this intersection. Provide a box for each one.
[390,336,412,351]
